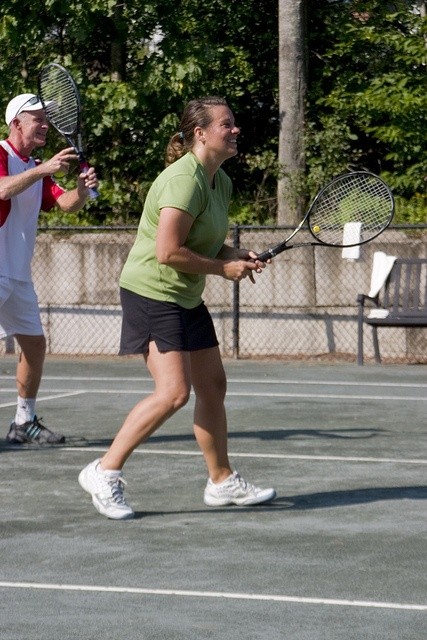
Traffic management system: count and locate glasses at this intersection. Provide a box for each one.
[14,95,41,119]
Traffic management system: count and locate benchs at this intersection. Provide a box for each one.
[357,253,427,367]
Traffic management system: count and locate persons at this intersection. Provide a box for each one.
[0,93,98,444]
[76,97,277,521]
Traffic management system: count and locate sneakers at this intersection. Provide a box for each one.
[203,470,276,507]
[77,458,135,520]
[7,415,65,445]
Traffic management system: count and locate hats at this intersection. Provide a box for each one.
[4,93,54,128]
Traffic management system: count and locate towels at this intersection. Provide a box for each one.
[340,222,364,259]
[367,251,397,298]
[367,309,390,319]
